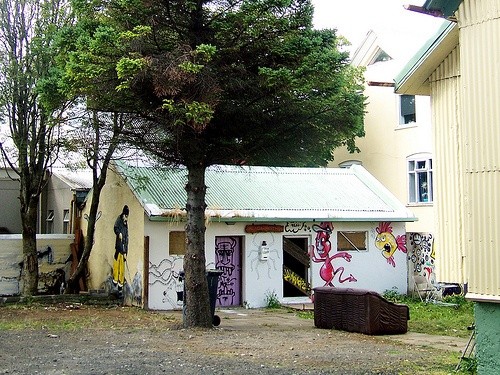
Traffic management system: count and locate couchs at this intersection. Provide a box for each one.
[312,287,409,337]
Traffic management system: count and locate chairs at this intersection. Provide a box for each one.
[412,275,438,304]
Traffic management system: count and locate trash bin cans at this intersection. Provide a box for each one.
[178,269,223,327]
[465,291,500,375]
